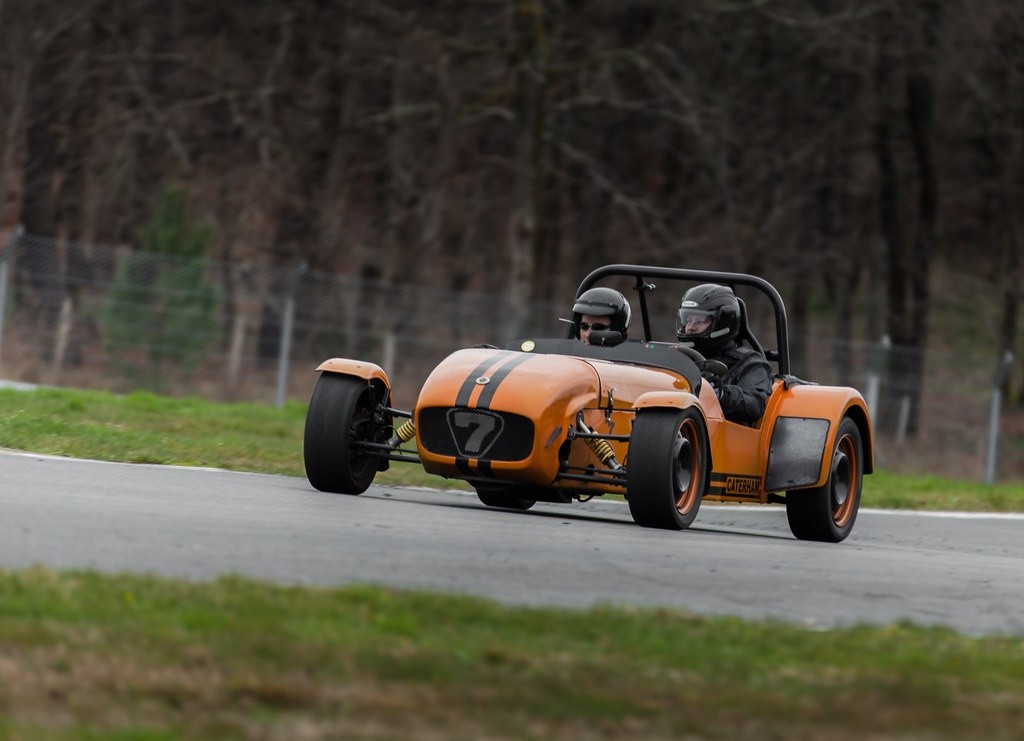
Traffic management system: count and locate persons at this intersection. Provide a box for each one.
[675,284,773,427]
[573,287,632,345]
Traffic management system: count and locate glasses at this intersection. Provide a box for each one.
[580,321,612,332]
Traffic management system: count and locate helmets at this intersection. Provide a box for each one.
[572,287,632,336]
[680,285,741,354]
